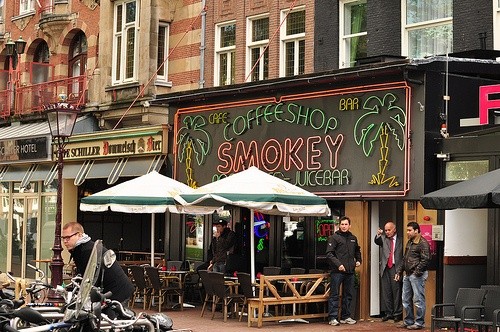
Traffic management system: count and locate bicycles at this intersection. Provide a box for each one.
[43,93,81,307]
[0,264,194,332]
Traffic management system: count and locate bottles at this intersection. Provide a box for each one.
[257,271,261,278]
[233,271,238,277]
[161,264,177,271]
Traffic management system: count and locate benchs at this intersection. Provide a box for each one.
[247,273,343,327]
[226,273,261,320]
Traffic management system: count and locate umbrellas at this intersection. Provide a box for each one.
[173,166,332,318]
[80,169,222,306]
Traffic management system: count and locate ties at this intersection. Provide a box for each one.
[387,237,395,269]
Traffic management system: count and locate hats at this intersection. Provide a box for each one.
[212,218,227,225]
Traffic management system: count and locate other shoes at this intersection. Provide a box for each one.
[396,322,408,328]
[407,324,426,330]
[382,315,393,321]
[394,316,403,322]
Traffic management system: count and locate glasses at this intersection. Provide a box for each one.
[59,232,80,240]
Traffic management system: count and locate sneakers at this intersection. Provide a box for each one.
[339,317,357,325]
[328,319,339,326]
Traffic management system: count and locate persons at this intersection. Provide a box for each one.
[326,216,362,326]
[374,222,432,330]
[208,219,235,312]
[62,222,136,303]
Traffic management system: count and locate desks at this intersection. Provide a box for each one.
[157,271,198,314]
[277,280,312,324]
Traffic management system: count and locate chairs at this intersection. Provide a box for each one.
[430,285,500,332]
[118,265,328,323]
